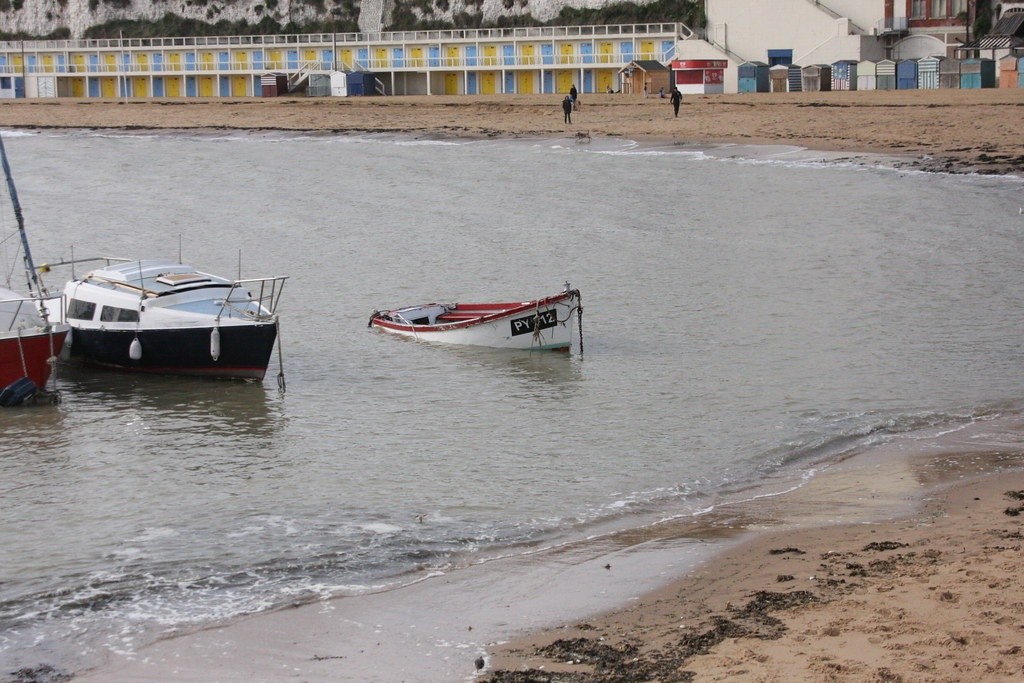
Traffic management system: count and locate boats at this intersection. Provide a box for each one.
[18,233,293,392]
[0,135,73,409]
[367,279,586,361]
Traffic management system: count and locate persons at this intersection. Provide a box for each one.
[659,87,667,98]
[563,96,571,124]
[606,84,612,93]
[570,85,577,106]
[670,87,682,117]
[644,83,648,98]
